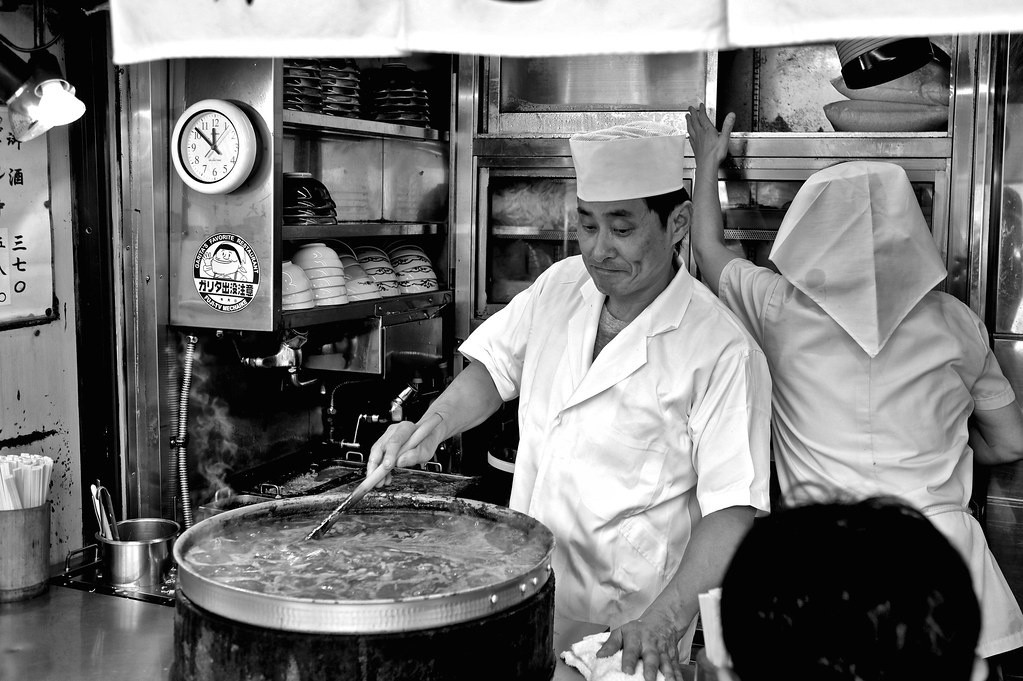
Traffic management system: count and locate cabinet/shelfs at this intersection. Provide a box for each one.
[460,32,1023,520]
[170,47,457,333]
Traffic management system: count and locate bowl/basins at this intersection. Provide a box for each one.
[282,171,338,225]
[283,58,431,129]
[281,242,440,310]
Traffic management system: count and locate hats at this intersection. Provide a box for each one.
[570,119,685,202]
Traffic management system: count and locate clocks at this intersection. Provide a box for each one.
[171,99,263,196]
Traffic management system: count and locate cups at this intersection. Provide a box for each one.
[0,501,51,604]
[95,517,181,588]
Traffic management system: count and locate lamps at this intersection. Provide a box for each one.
[0,0,87,142]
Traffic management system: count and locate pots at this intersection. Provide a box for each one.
[172,494,559,680]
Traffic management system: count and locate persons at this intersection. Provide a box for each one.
[719,481,983,681]
[368,122,772,681]
[685,103,1023,681]
